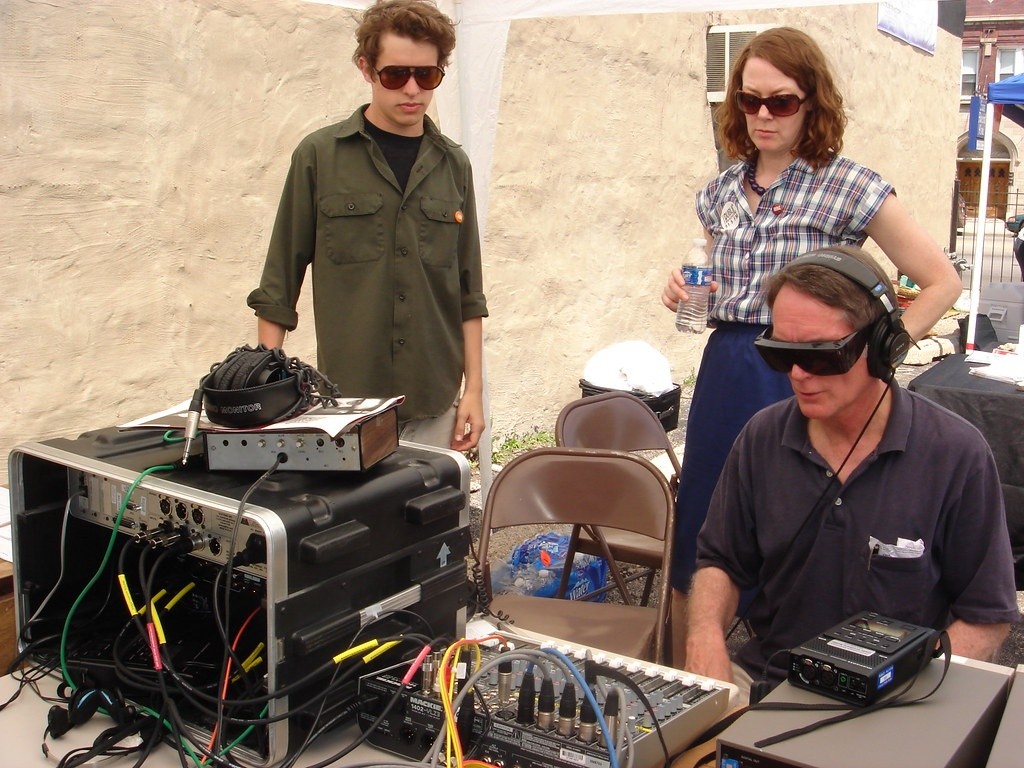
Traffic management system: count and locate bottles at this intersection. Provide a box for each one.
[490,533,606,602]
[675,239,713,334]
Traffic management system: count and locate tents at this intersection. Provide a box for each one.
[966,73,1024,352]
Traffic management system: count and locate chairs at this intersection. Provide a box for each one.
[554,393,682,608]
[479,445,670,666]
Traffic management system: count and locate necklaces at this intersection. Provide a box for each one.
[748,148,770,197]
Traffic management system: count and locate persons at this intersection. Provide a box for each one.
[661,26,963,668]
[683,242,1020,716]
[247,1,493,455]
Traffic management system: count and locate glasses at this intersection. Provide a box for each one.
[735,86,807,117]
[373,65,445,90]
[754,325,870,376]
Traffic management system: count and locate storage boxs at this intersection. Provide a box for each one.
[578,378,682,433]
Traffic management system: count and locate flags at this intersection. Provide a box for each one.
[967,96,980,149]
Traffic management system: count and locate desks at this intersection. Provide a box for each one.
[0,667,416,768]
[907,353,1024,592]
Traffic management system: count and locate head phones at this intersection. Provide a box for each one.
[205,343,319,428]
[778,251,912,379]
